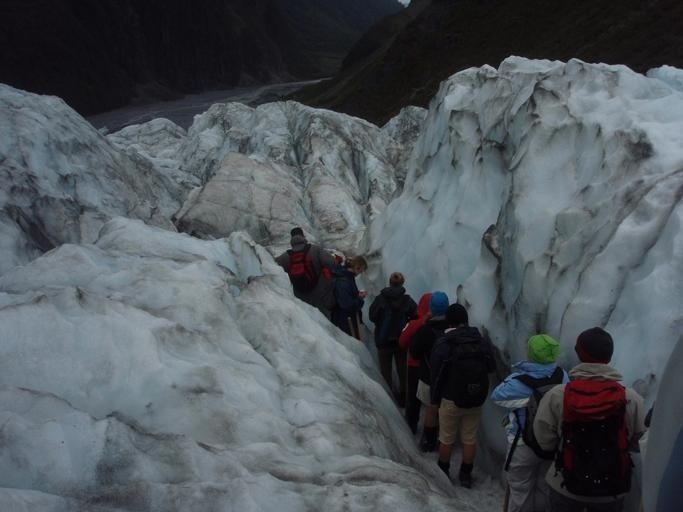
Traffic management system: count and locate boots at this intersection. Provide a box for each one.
[420,426,438,452]
[438,461,474,487]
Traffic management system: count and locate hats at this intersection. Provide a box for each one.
[418,291,469,326]
[389,272,405,286]
[526,333,559,364]
[575,326,614,364]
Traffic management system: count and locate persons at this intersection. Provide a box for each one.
[327,254,367,338]
[405,291,451,453]
[366,270,418,408]
[274,229,340,324]
[491,332,567,511]
[427,302,495,489]
[531,326,646,511]
[397,291,436,435]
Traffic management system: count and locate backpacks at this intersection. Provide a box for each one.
[287,245,318,291]
[376,293,411,349]
[311,264,336,310]
[558,380,633,495]
[445,331,490,409]
[511,365,563,458]
